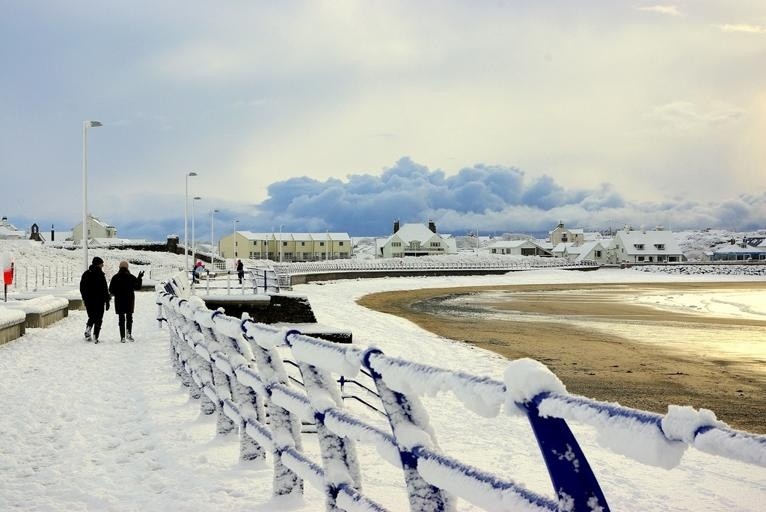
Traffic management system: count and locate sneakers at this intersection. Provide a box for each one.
[120,337,126,343]
[94,339,100,344]
[84,330,92,341]
[126,334,134,342]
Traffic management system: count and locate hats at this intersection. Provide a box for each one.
[91,256,103,265]
[118,261,128,269]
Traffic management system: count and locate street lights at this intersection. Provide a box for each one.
[279,224,285,266]
[211,209,220,270]
[184,172,197,278]
[192,196,201,270]
[234,220,239,270]
[82,120,102,273]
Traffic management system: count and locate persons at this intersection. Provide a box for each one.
[193,261,206,281]
[79,256,110,344]
[236,259,243,284]
[108,260,145,343]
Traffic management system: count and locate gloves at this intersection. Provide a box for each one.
[138,270,145,278]
